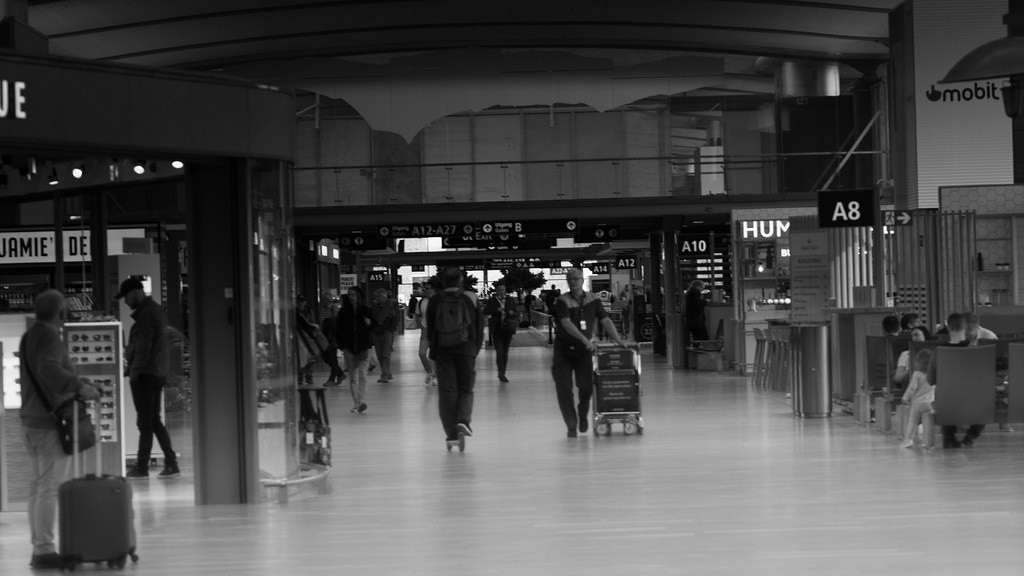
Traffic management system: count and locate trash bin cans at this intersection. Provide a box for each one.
[792,323,832,417]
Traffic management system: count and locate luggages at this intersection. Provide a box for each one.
[57,384,141,569]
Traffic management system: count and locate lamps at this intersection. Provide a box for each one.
[72,165,85,178]
[49,168,58,185]
[133,160,145,175]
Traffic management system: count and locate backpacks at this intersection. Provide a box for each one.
[434,287,472,348]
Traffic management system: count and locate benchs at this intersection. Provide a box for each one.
[861,335,1024,451]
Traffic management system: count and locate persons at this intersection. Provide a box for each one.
[551,268,628,438]
[882,311,1000,448]
[114,277,180,480]
[295,293,313,385]
[409,281,440,387]
[685,281,711,347]
[520,291,536,324]
[482,283,521,383]
[548,285,561,313]
[318,290,346,387]
[372,288,400,384]
[426,267,484,445]
[19,290,103,568]
[596,282,629,341]
[335,286,376,413]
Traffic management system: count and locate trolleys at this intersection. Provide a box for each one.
[593,341,644,437]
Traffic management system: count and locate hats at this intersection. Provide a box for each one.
[114,278,144,299]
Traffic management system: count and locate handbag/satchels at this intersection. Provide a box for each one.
[60,396,96,456]
[297,312,330,368]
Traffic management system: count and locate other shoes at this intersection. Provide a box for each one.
[962,435,975,446]
[498,372,509,382]
[334,372,347,385]
[323,378,336,387]
[30,550,64,569]
[922,443,935,449]
[446,433,459,444]
[943,435,961,449]
[425,371,437,386]
[568,428,577,438]
[579,415,588,432]
[457,420,472,436]
[126,466,149,479]
[158,466,180,477]
[376,374,394,383]
[901,438,915,448]
[368,363,376,371]
[351,403,367,412]
